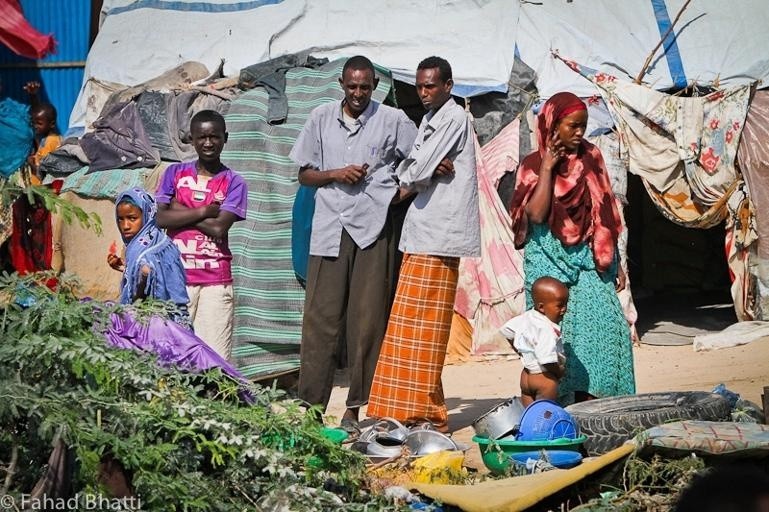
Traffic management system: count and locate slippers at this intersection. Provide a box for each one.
[338,418,360,445]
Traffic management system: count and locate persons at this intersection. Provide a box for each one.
[106,185,193,333]
[155,108,248,364]
[286,54,420,444]
[507,93,636,406]
[23,78,63,272]
[498,275,569,411]
[368,54,483,444]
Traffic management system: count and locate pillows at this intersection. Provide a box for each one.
[631,418,768,463]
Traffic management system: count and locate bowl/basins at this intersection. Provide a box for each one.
[260,396,587,473]
